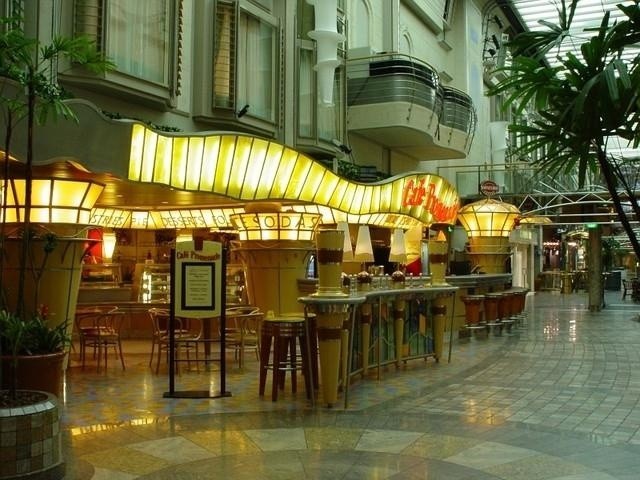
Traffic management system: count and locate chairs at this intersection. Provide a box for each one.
[148,306,264,378]
[458,285,530,340]
[74,304,127,375]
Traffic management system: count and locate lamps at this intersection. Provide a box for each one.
[456,197,522,238]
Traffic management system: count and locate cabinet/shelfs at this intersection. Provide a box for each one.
[135,262,244,308]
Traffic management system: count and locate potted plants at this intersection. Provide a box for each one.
[0,307,72,399]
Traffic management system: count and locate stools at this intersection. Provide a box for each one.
[259,312,317,401]
